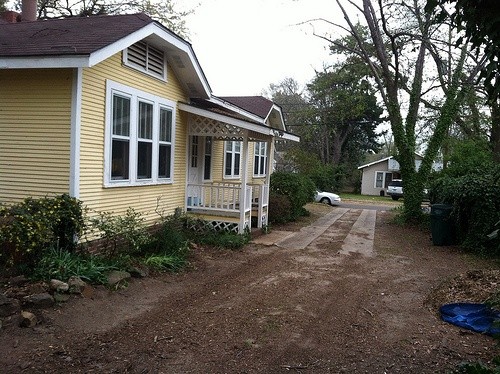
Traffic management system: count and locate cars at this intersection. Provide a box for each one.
[309,187,341,205]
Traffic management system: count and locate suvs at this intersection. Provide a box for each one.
[385,179,428,200]
